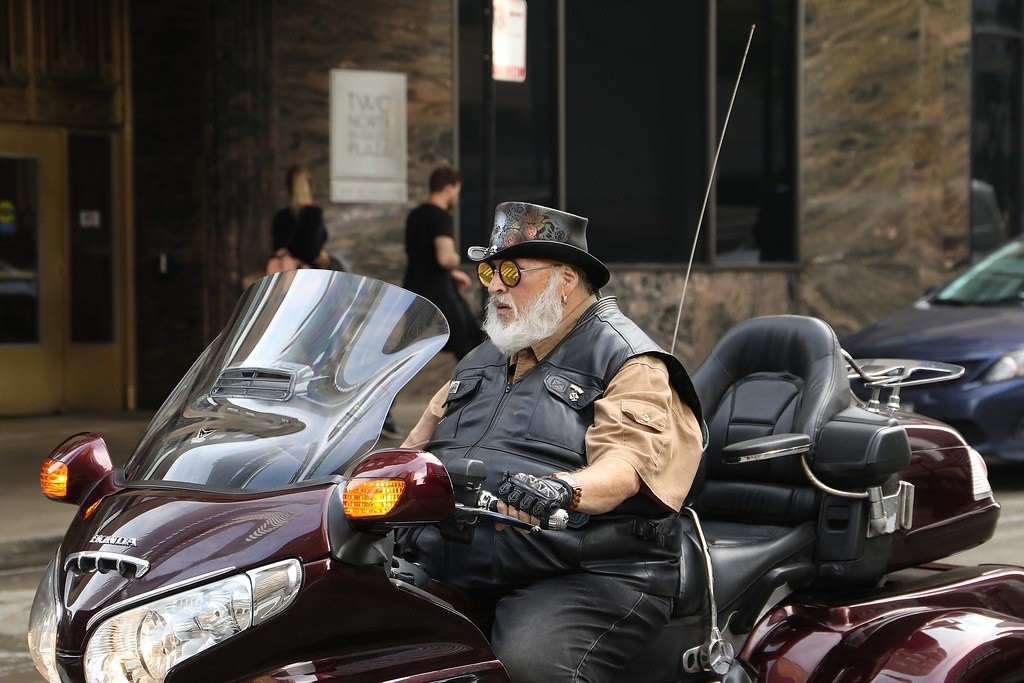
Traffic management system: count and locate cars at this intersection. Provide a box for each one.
[833,231,1023,471]
[966,179,1016,271]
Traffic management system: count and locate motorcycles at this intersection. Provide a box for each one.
[27,269,1024,682]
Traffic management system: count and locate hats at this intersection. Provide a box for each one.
[467,202,610,291]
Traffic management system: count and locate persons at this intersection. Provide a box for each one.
[265,165,330,275]
[398,165,712,683]
[376,167,488,439]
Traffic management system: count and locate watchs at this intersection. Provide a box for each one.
[551,471,583,513]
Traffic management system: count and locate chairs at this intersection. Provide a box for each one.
[692,314,855,616]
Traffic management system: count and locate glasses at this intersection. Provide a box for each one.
[476,259,562,288]
